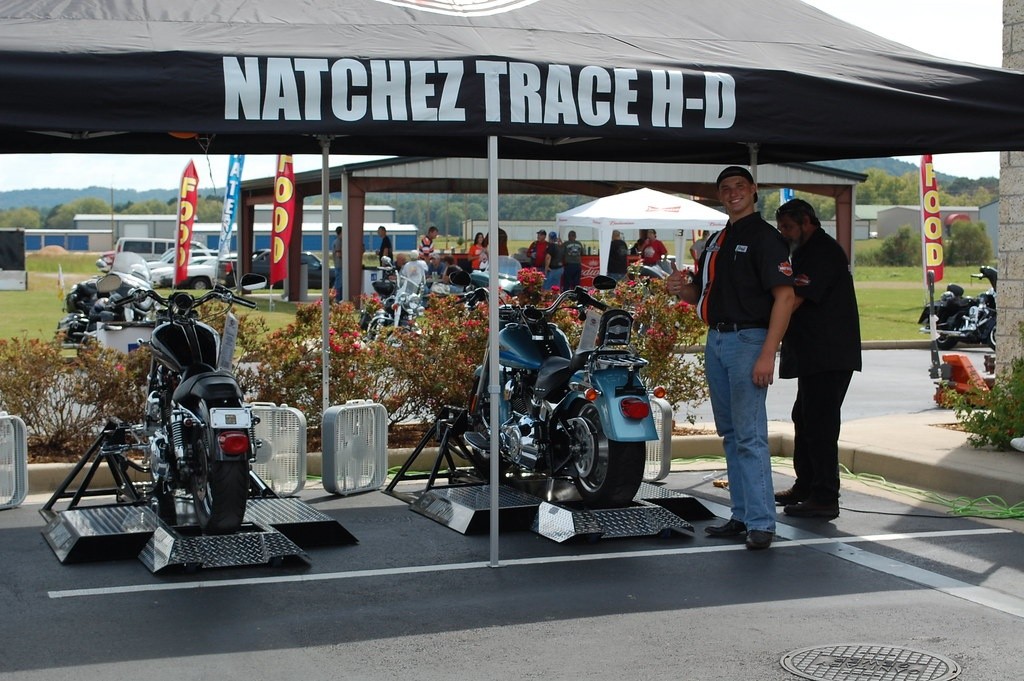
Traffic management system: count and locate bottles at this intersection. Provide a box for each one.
[592,246,596,255]
[450,247,455,254]
[584,245,591,255]
[627,243,632,255]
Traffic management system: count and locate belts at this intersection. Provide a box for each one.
[709,321,770,333]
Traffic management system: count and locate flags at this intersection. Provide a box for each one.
[174,161,199,285]
[269,155,296,284]
[220,154,245,255]
[920,154,945,289]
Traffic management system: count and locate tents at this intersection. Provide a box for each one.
[554,186,730,293]
[0,0,1023,567]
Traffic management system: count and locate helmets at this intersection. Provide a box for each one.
[941,291,955,302]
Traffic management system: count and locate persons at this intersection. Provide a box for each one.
[667,166,794,551]
[526,230,583,292]
[689,230,710,261]
[377,226,393,266]
[392,253,408,270]
[632,229,669,266]
[443,255,463,284]
[419,226,438,264]
[426,253,445,276]
[607,230,628,281]
[332,226,342,304]
[773,197,862,519]
[467,225,509,272]
[401,250,430,308]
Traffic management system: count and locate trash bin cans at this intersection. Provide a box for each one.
[360,266,384,309]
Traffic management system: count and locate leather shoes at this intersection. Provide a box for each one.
[704,518,746,537]
[746,530,774,549]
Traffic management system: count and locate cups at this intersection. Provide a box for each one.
[440,249,444,253]
[460,249,465,253]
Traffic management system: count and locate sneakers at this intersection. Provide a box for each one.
[774,487,808,505]
[784,499,841,518]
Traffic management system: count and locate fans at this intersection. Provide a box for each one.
[321,397,389,497]
[251,400,308,498]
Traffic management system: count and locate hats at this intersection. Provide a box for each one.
[549,232,557,239]
[536,230,547,236]
[717,166,758,204]
[410,250,420,260]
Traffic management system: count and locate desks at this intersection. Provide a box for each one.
[437,252,469,264]
[561,254,641,293]
